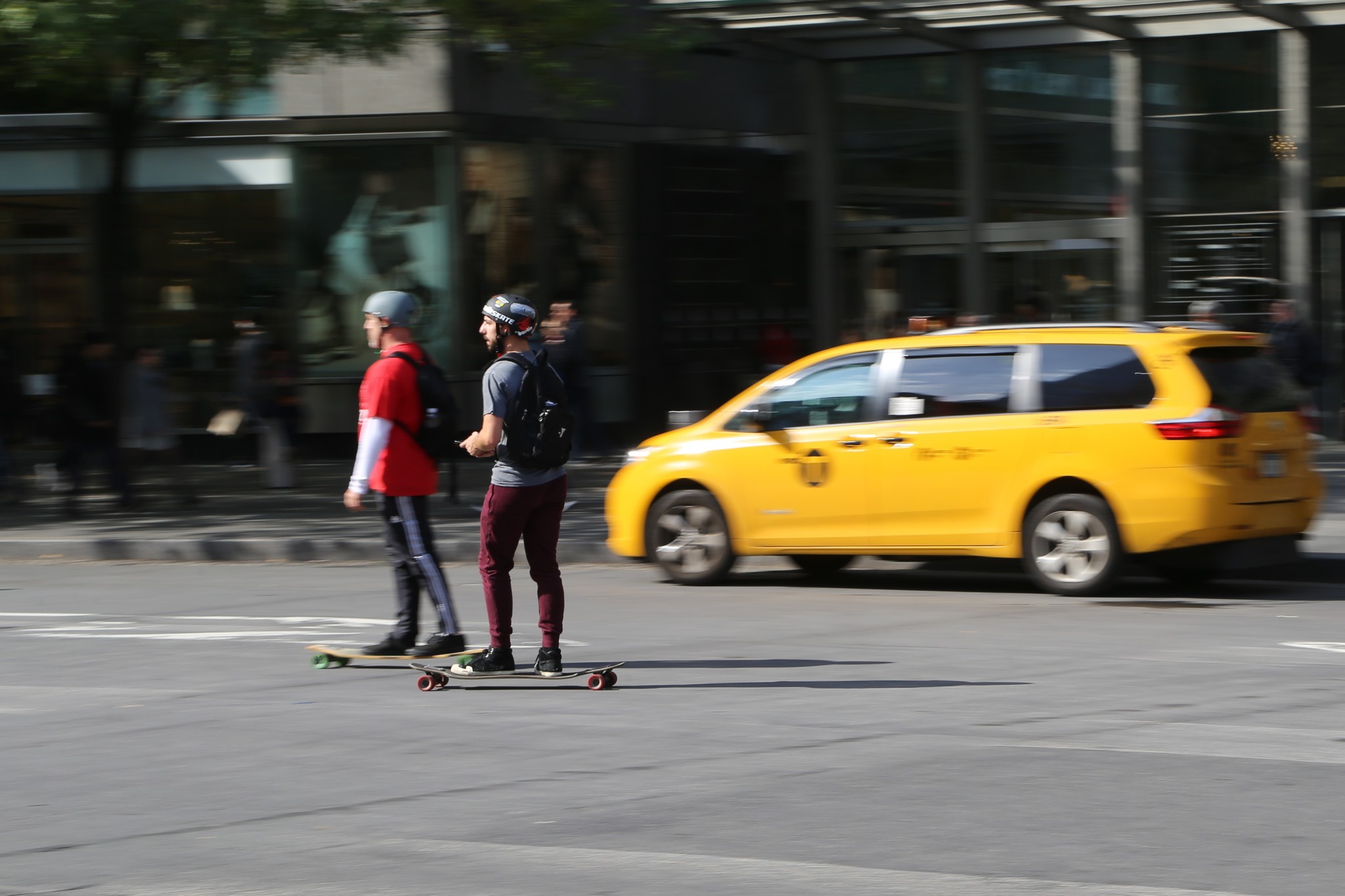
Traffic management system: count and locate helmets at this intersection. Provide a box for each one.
[481,294,538,338]
[362,291,419,330]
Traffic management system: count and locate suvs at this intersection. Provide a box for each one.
[605,320,1324,596]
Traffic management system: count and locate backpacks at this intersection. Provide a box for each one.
[384,351,464,462]
[493,348,572,469]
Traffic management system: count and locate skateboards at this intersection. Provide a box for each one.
[405,656,627,691]
[303,643,498,671]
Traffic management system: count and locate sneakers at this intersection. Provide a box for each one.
[363,631,418,656]
[404,632,465,657]
[450,645,516,675]
[534,648,563,677]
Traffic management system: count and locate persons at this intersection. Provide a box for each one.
[1,323,193,518]
[449,293,577,677]
[343,291,466,657]
[1189,296,1322,406]
